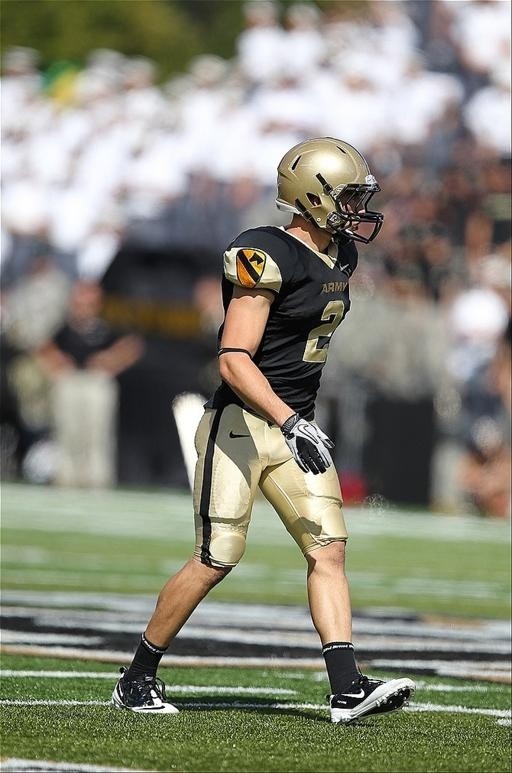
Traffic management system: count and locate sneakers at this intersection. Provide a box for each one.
[109,664,178,715]
[324,674,416,726]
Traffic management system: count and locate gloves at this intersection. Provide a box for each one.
[280,409,336,478]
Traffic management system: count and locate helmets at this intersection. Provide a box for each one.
[273,132,386,246]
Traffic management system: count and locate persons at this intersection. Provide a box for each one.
[111,137,416,723]
